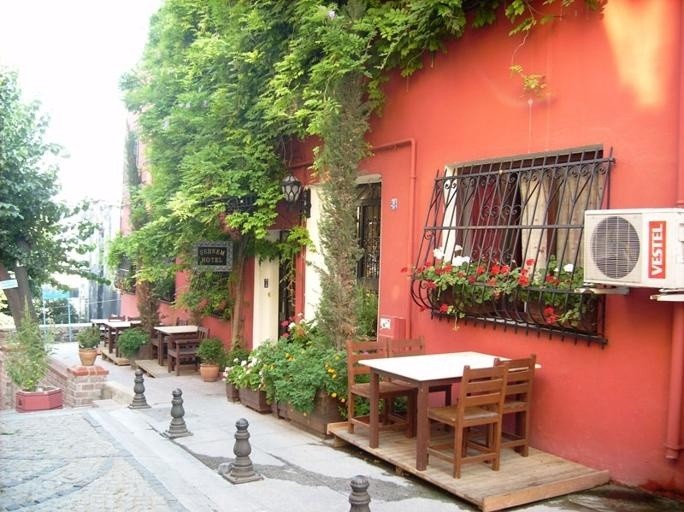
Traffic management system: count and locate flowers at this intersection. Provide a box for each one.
[222,312,349,414]
[396,245,599,330]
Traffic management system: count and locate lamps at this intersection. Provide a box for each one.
[280,170,306,213]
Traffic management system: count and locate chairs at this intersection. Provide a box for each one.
[343,334,536,480]
[104,314,210,377]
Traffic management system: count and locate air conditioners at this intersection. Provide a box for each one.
[583,207,684,290]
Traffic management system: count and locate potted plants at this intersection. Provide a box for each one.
[196,339,223,382]
[3,293,64,412]
[78,328,101,366]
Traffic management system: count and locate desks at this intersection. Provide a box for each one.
[90,318,108,348]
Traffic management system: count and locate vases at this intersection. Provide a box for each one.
[271,391,343,439]
